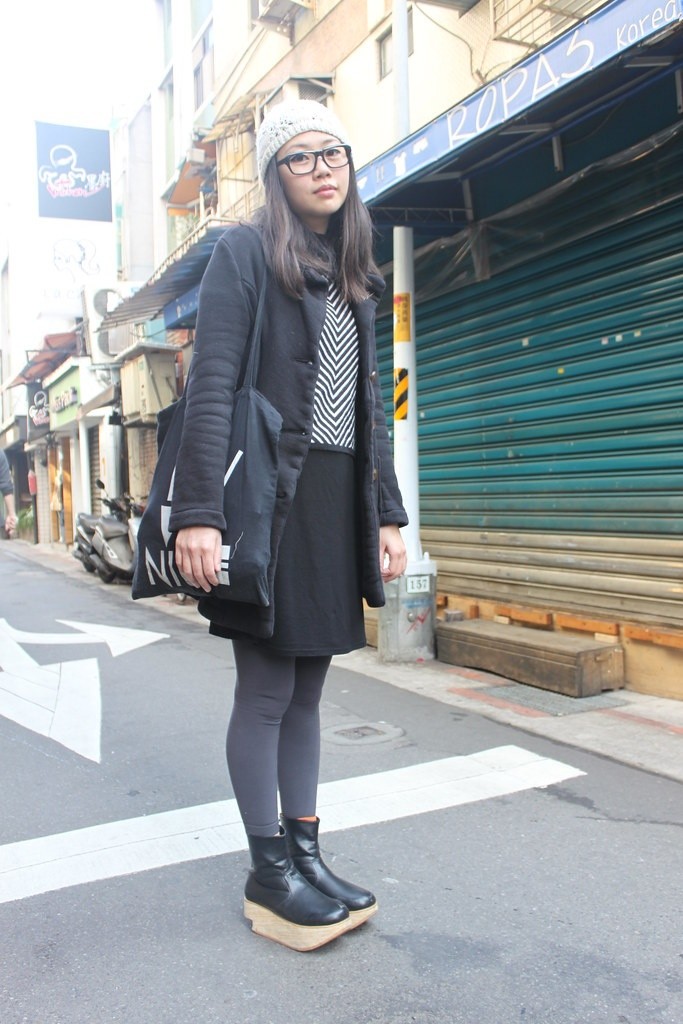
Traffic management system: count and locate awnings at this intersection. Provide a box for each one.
[93,216,239,334]
[6,331,77,391]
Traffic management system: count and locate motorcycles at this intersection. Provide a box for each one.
[87,492,150,584]
[71,477,130,573]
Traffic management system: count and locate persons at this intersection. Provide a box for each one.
[169,97,410,949]
[0,447,18,535]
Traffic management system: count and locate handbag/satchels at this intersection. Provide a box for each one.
[130,220,283,610]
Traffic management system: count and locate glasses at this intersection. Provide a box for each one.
[276,143,352,175]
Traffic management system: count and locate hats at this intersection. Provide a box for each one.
[256,98,349,186]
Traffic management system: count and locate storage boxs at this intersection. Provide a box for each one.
[436,619,626,698]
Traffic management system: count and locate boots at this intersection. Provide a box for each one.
[280,814,378,926]
[244,825,353,952]
